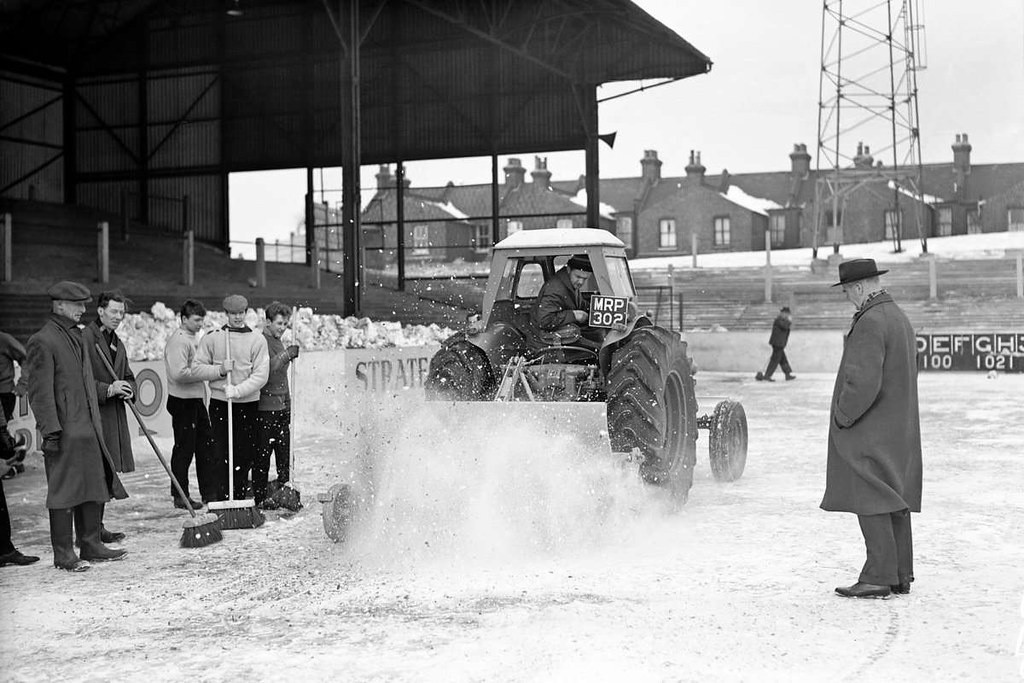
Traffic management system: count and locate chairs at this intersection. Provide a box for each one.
[508,304,594,363]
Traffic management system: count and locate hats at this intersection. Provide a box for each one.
[830,259,889,288]
[223,295,248,312]
[567,254,593,272]
[781,307,790,312]
[48,281,93,303]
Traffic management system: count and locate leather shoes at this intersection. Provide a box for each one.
[891,581,910,594]
[835,582,891,599]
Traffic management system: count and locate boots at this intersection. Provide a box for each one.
[75,503,128,561]
[50,509,88,572]
[100,504,125,542]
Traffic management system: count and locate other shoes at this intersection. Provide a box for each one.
[174,497,202,510]
[2,463,25,479]
[764,375,775,382]
[785,374,795,380]
[0,551,40,566]
[258,496,279,510]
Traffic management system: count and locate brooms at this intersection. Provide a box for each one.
[206,328,266,530]
[94,343,224,549]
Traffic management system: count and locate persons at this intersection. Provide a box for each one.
[764,308,796,381]
[164,295,299,509]
[819,259,923,600]
[0,281,138,573]
[527,254,601,365]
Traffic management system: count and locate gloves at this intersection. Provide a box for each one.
[287,345,299,361]
[107,380,134,399]
[41,432,60,458]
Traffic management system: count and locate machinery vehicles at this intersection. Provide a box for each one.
[424,229,749,514]
